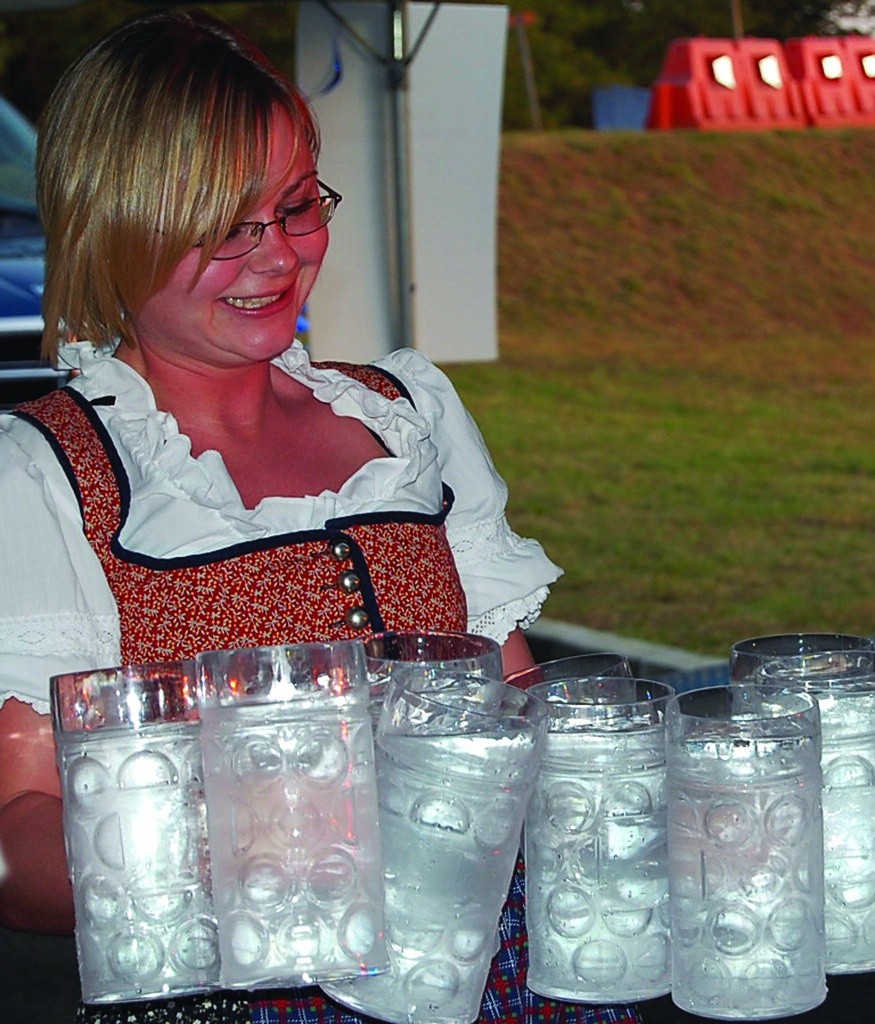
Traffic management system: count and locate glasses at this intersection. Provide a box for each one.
[152,177,342,262]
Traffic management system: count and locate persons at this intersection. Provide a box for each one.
[0,7,642,1024]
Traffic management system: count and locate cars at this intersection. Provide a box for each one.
[1,98,128,380]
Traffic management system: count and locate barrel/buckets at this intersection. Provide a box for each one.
[593,86,650,131]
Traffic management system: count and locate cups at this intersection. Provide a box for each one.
[505,652,635,692]
[51,659,222,1004]
[196,638,392,991]
[667,685,827,1021]
[319,669,552,1024]
[526,678,676,1004]
[365,631,503,736]
[758,649,875,975]
[731,633,874,690]
